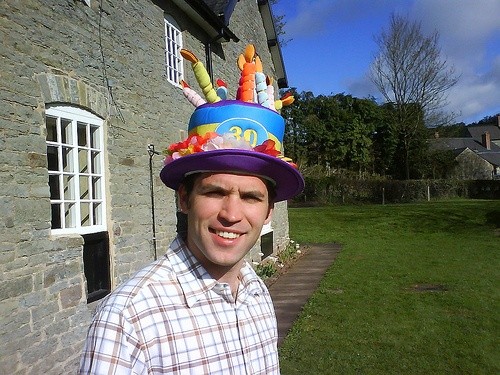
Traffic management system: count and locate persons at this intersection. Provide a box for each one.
[74,48,306,375]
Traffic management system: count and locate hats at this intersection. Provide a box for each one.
[158,43,305,203]
